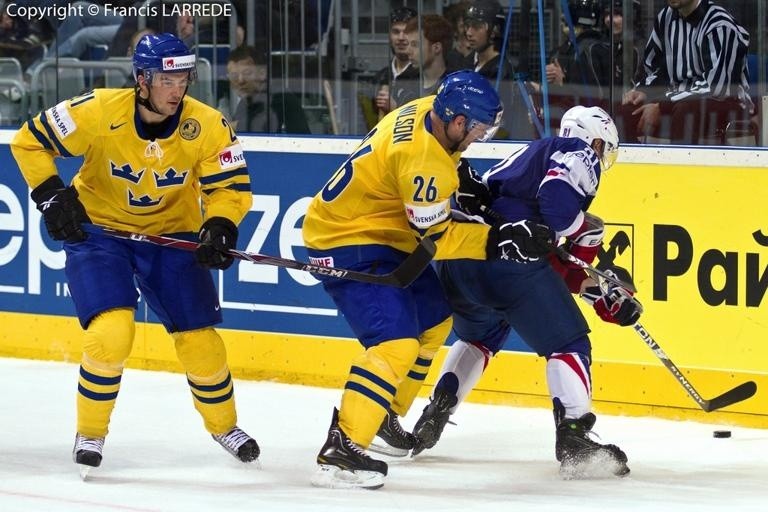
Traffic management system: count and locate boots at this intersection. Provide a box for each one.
[551,396,631,482]
[367,408,416,457]
[210,423,266,471]
[412,372,459,458]
[310,406,389,491]
[71,431,106,484]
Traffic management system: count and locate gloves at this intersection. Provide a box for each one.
[194,215,240,271]
[588,276,647,327]
[494,217,556,263]
[30,174,94,245]
[551,213,612,292]
[453,157,495,216]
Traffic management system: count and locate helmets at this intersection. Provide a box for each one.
[431,68,503,142]
[604,0,641,20]
[557,104,620,171]
[462,0,507,29]
[131,32,198,88]
[561,0,600,28]
[388,6,416,23]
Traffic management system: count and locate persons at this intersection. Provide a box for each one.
[301,68,506,479]
[445,4,472,70]
[534,1,644,143]
[9,32,261,466]
[0,0,194,104]
[620,0,755,144]
[224,45,311,134]
[373,7,418,122]
[396,14,458,108]
[463,0,514,139]
[413,104,643,476]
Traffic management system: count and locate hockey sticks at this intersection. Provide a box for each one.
[583,264,758,413]
[75,221,437,292]
[550,244,636,294]
